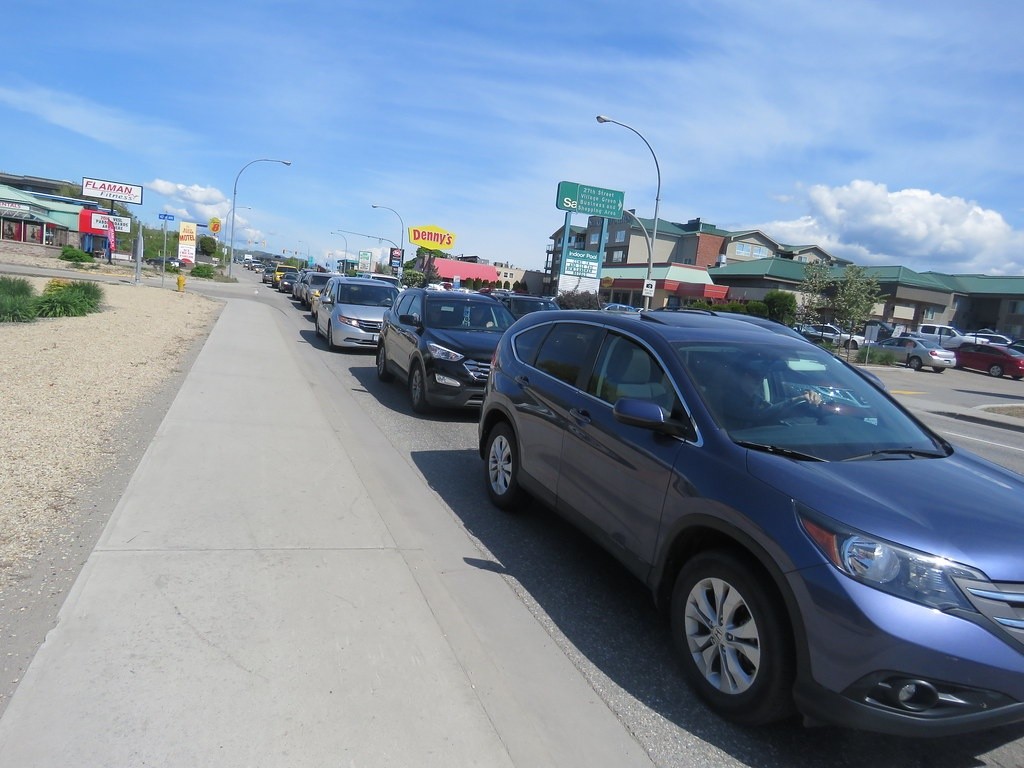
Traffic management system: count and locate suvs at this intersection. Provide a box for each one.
[360,273,406,293]
[480,309,1024,737]
[916,323,989,351]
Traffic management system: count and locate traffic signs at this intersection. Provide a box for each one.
[557,180,626,222]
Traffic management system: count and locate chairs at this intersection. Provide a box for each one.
[607,348,666,404]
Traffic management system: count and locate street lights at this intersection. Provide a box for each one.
[222,206,252,264]
[336,204,404,286]
[595,115,664,312]
[229,158,291,282]
[330,232,348,277]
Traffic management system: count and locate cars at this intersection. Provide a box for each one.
[965,329,1024,353]
[861,336,958,373]
[808,323,874,350]
[234,258,344,312]
[949,343,1024,379]
[374,289,523,413]
[146,257,186,269]
[316,276,401,349]
[601,303,655,314]
[788,322,841,347]
[860,319,921,344]
[650,307,888,415]
[424,280,562,321]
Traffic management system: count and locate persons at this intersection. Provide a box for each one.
[725,366,822,433]
[469,308,494,328]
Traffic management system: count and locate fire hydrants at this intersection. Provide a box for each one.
[176,276,187,293]
